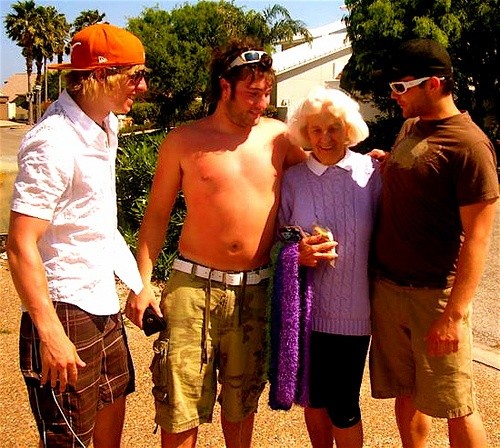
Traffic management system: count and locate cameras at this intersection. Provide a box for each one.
[142,305,167,337]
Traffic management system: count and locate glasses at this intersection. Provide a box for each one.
[389,77,445,94]
[219,50,268,79]
[106,66,146,83]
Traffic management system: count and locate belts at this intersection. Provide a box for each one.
[171,258,274,287]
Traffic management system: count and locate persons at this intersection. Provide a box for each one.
[279,88,382,448]
[6,23,143,448]
[125,38,307,448]
[368,38,500,448]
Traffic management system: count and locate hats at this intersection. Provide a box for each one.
[46,21,146,71]
